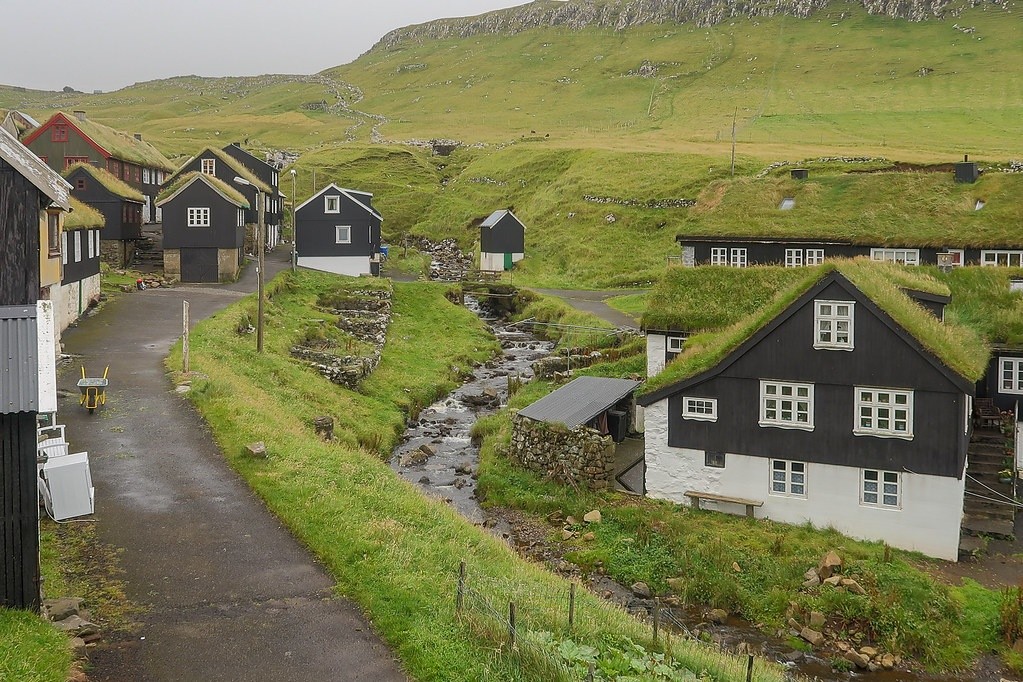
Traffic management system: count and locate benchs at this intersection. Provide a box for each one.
[683,491,764,518]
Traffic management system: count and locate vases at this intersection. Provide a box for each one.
[999,478,1011,484]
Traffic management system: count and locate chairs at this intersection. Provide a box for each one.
[37,424,70,457]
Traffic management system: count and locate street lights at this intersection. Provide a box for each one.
[234,176,263,353]
[290,169,298,272]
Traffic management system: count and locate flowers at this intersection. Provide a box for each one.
[998,469,1011,479]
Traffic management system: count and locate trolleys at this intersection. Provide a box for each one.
[78,364,110,415]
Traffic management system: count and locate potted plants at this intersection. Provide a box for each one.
[1003,441,1014,455]
[1005,425,1014,437]
